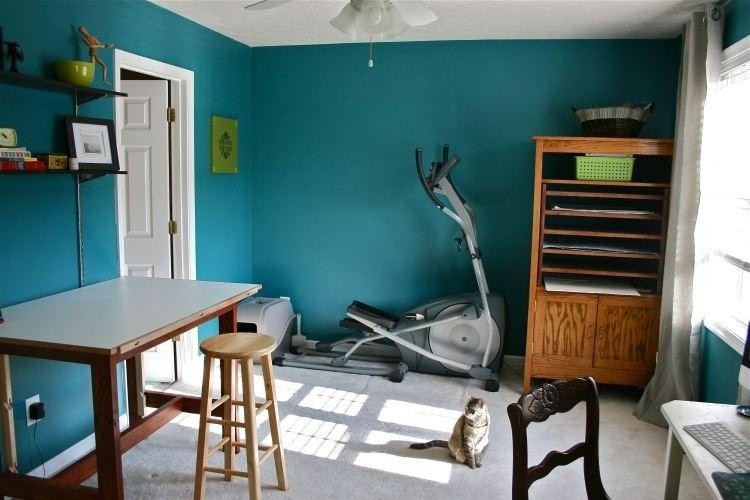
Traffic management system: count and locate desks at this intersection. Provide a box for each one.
[1,275,263,500]
[660,399,750,500]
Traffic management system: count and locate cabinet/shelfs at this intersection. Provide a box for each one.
[523,135,675,395]
[0,69,129,183]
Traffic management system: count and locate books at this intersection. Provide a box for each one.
[0,146,47,172]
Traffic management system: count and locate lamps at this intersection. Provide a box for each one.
[355,0,391,68]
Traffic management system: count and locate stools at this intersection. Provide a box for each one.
[193,332,289,500]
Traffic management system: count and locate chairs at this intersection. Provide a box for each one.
[507,376,612,500]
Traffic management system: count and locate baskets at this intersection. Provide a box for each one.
[570,102,655,138]
[572,155,637,181]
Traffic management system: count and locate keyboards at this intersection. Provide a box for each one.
[683,421,750,474]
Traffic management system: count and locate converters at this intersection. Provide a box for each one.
[30,402,45,419]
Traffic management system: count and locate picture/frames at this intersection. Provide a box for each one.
[64,115,120,171]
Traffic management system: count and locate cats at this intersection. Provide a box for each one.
[410,391,491,470]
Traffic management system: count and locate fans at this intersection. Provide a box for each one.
[244,0,438,36]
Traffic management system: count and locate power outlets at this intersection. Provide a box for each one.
[25,394,42,427]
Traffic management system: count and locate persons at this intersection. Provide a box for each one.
[79,27,113,85]
